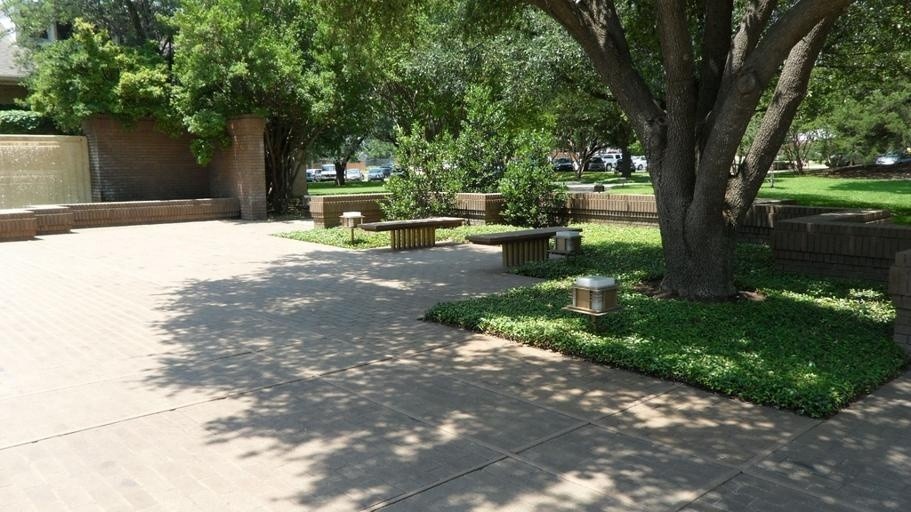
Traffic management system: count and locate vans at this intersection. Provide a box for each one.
[602,152,621,170]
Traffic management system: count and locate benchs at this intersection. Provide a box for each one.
[466,229,556,268]
[360,215,466,248]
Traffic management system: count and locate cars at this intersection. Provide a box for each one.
[305,163,408,183]
[633,156,647,171]
[876,150,911,166]
[554,155,604,172]
